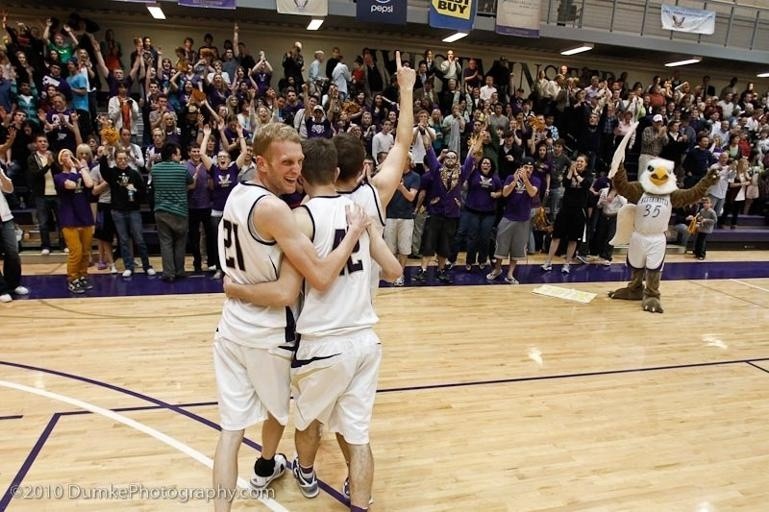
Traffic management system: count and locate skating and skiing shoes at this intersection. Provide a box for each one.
[63,257,226,296]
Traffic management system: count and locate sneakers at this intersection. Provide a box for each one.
[479,262,486,272]
[415,267,432,286]
[0,293,13,303]
[248,450,289,493]
[464,263,474,273]
[446,259,456,272]
[39,247,52,256]
[343,475,376,506]
[12,283,30,296]
[432,269,454,285]
[392,271,408,287]
[559,262,572,274]
[485,268,503,280]
[575,252,612,266]
[504,276,520,285]
[539,262,554,272]
[290,454,323,499]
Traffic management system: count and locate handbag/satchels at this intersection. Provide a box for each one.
[687,211,701,235]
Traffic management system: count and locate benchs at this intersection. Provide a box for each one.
[670,211,768,225]
[674,223,769,245]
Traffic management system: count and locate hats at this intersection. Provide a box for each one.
[521,156,536,166]
[652,113,664,123]
[312,104,327,115]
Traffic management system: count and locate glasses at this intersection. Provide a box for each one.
[444,155,458,160]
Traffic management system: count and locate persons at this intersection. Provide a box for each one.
[299,49,416,505]
[220,137,404,512]
[212,122,372,512]
